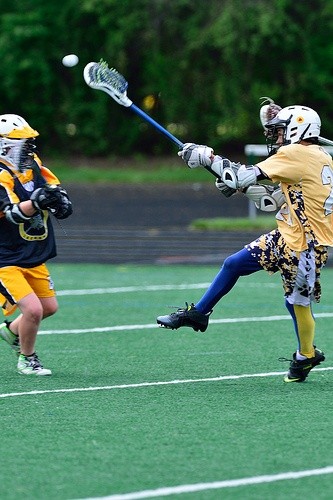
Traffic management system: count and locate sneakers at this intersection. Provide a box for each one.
[16,354,52,376]
[0,320,20,354]
[156,301,213,332]
[277,344,326,383]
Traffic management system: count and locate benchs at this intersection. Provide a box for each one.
[245,145,333,218]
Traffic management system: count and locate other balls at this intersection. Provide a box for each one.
[62,54,79,68]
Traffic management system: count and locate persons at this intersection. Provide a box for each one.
[0,114,73,376]
[156,104,333,383]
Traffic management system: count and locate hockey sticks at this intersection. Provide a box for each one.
[83,61,225,182]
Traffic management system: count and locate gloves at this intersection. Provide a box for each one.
[177,140,214,169]
[215,178,239,199]
[48,187,73,220]
[29,186,61,210]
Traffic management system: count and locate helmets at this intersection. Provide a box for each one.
[264,105,322,156]
[0,114,40,170]
